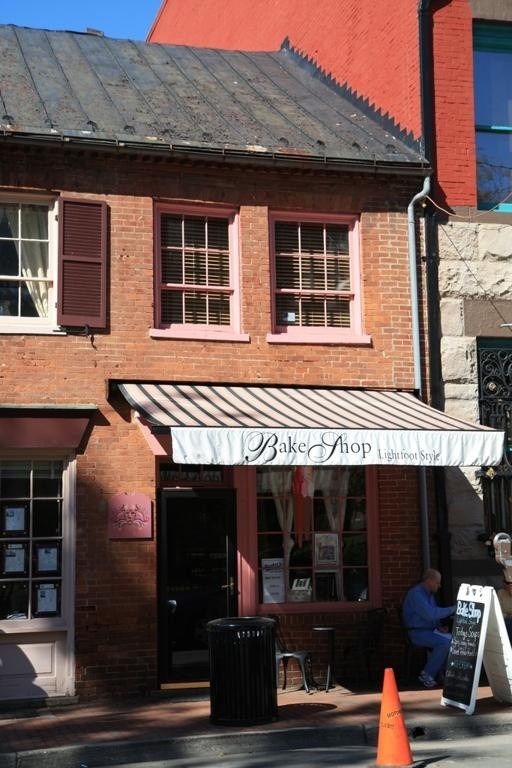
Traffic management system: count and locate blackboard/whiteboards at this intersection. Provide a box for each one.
[442,583,492,710]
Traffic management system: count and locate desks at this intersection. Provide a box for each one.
[310,624,372,696]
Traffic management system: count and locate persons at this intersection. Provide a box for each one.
[401,567,456,690]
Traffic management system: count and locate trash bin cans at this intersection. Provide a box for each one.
[205,616,278,727]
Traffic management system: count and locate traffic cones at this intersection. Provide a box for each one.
[367,667,427,768]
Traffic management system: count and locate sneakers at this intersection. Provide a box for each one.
[417,669,440,689]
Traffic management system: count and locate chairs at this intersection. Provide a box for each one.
[341,609,387,694]
[266,614,320,693]
[398,607,441,688]
